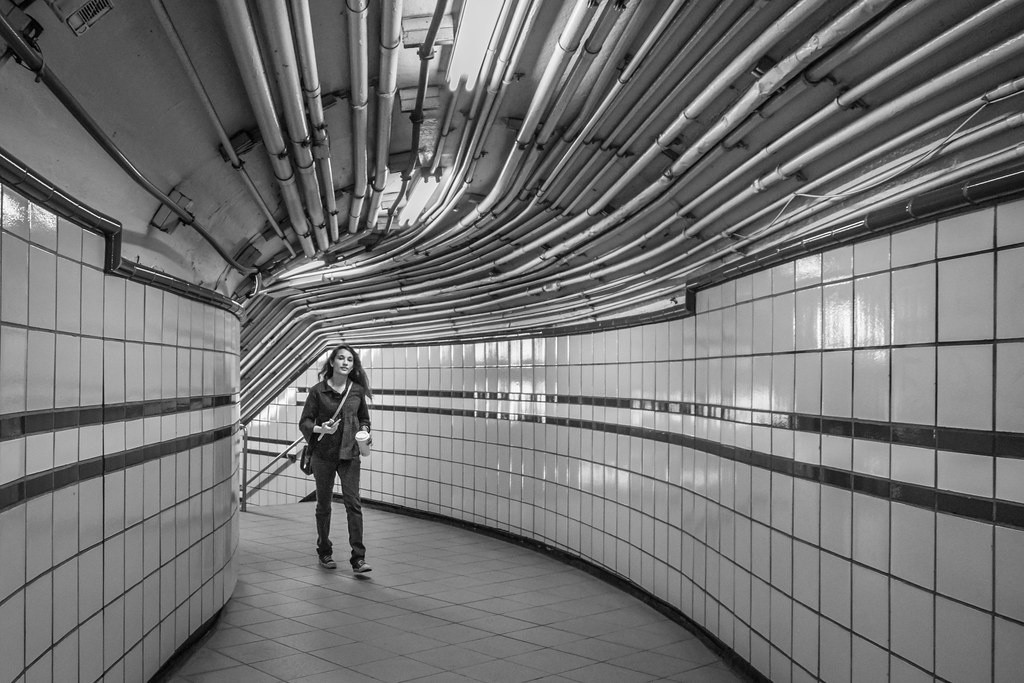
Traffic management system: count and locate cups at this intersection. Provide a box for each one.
[355,431,370,457]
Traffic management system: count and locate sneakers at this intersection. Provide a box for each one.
[319,555,336,568]
[354,559,372,572]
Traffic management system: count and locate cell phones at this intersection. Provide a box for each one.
[330,418,342,428]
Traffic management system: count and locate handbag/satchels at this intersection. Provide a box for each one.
[300,444,313,475]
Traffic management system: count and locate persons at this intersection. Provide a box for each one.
[299,345,372,573]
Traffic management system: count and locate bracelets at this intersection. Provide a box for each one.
[359,425,370,432]
